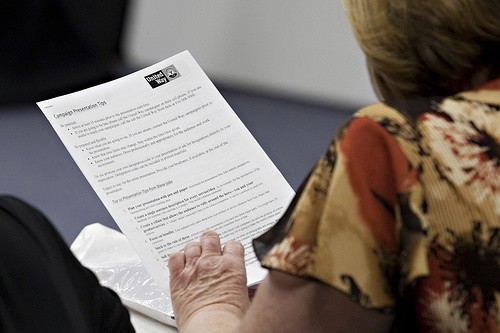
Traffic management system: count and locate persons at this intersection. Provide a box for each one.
[167,1,499,332]
